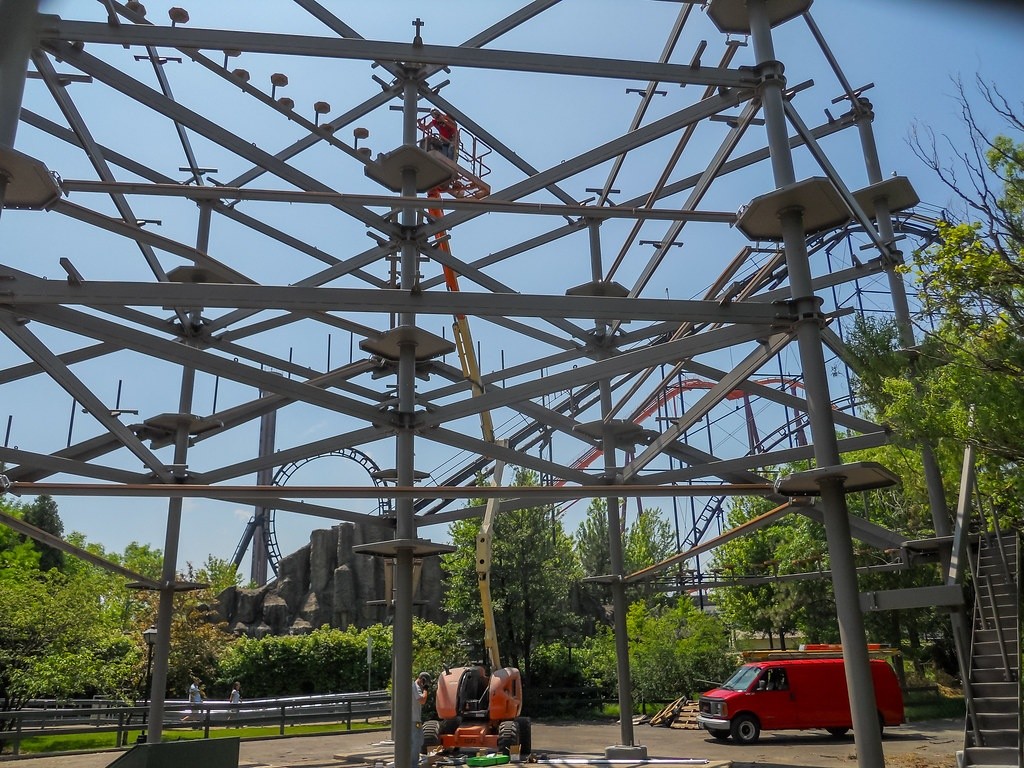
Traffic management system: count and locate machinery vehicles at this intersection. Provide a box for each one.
[416,665,531,758]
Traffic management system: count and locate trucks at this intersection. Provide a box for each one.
[694,643,905,744]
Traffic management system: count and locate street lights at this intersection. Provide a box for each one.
[136,624,158,743]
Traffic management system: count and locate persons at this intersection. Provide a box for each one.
[182,678,201,730]
[226,682,242,729]
[412,672,431,768]
[417,109,461,165]
